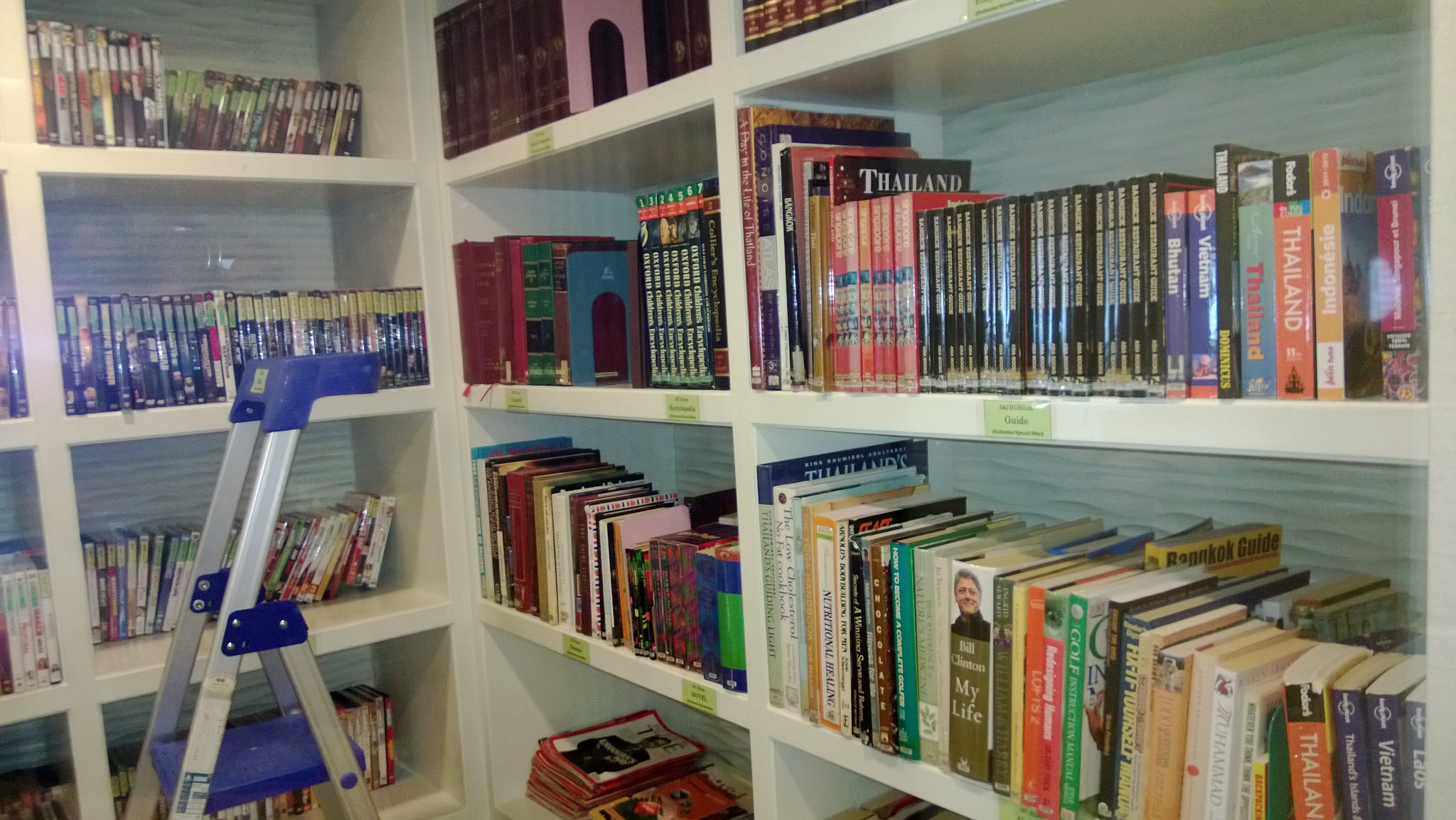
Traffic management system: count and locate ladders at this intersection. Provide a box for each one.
[123,353,379,820]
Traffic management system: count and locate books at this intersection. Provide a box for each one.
[452,175,731,390]
[525,710,752,820]
[754,439,1429,820]
[0,492,397,701]
[469,438,748,693]
[734,97,1432,401]
[433,0,886,162]
[0,684,396,820]
[23,19,362,158]
[0,287,431,420]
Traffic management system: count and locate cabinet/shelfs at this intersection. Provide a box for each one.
[3,0,1456,819]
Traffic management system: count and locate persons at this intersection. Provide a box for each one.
[951,569,991,641]
[862,549,870,579]
[560,735,671,774]
